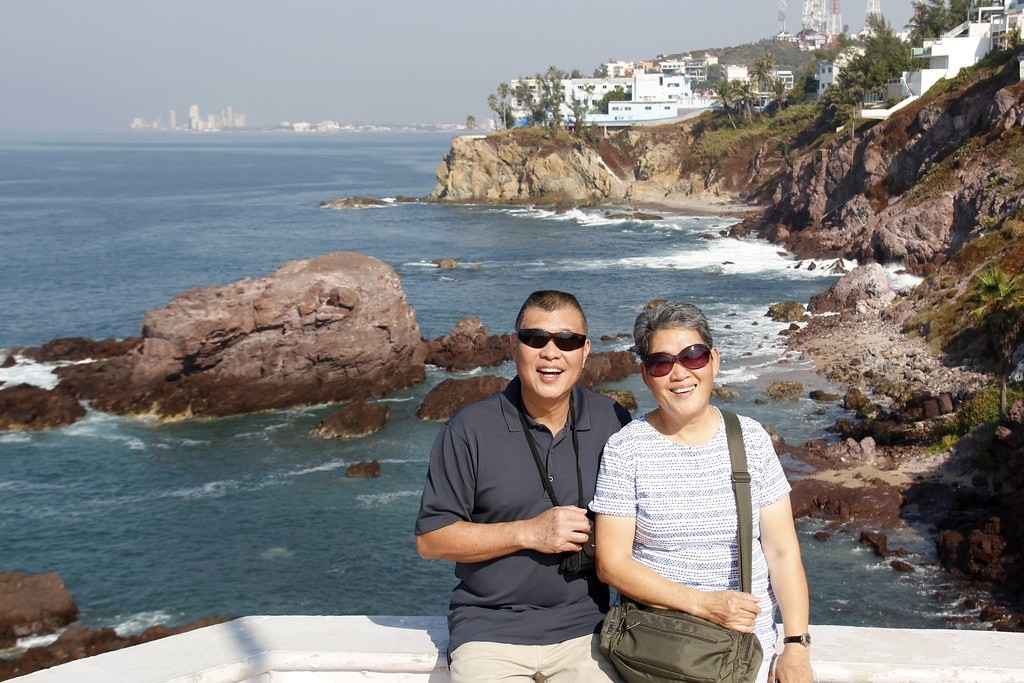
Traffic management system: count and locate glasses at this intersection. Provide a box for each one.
[643,344,711,377]
[514,328,587,351]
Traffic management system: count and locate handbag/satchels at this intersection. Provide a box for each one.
[597,598,765,683]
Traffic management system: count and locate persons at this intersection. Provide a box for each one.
[414,290,632,683]
[588,301,817,683]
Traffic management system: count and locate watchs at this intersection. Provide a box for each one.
[783,633,811,649]
